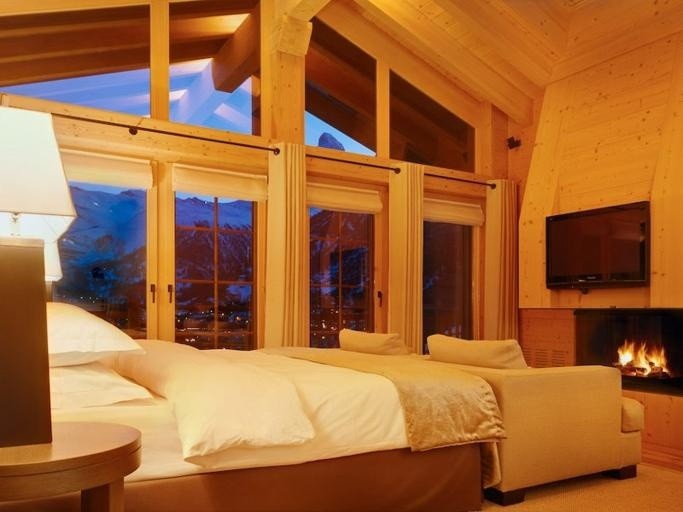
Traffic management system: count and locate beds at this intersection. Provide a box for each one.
[0,347,485,512]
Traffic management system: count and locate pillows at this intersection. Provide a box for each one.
[45,301,154,409]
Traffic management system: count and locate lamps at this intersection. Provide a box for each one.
[0,105,75,240]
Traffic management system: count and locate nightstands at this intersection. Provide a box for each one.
[0,422,143,512]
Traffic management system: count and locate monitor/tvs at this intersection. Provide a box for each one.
[546,201,651,289]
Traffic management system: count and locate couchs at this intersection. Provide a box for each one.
[337,329,645,506]
